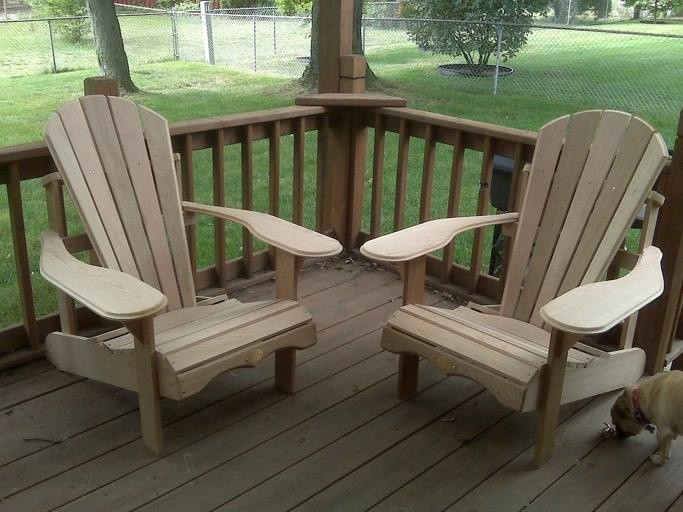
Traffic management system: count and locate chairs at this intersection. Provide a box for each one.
[37,91,345,457]
[360,105,666,470]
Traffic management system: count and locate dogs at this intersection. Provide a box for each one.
[610,369,683,466]
